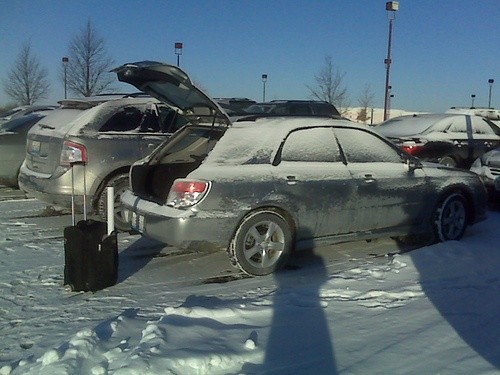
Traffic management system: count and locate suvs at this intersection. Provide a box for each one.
[15,92,241,233]
[244,100,340,117]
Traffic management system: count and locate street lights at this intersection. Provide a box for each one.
[262,75,267,103]
[62,58,68,100]
[383,2,399,123]
[488,78,495,108]
[471,95,475,107]
[175,43,182,67]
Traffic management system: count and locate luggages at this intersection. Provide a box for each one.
[63,161,119,292]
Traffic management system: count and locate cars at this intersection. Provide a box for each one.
[372,113,500,170]
[0,111,58,190]
[110,60,488,276]
